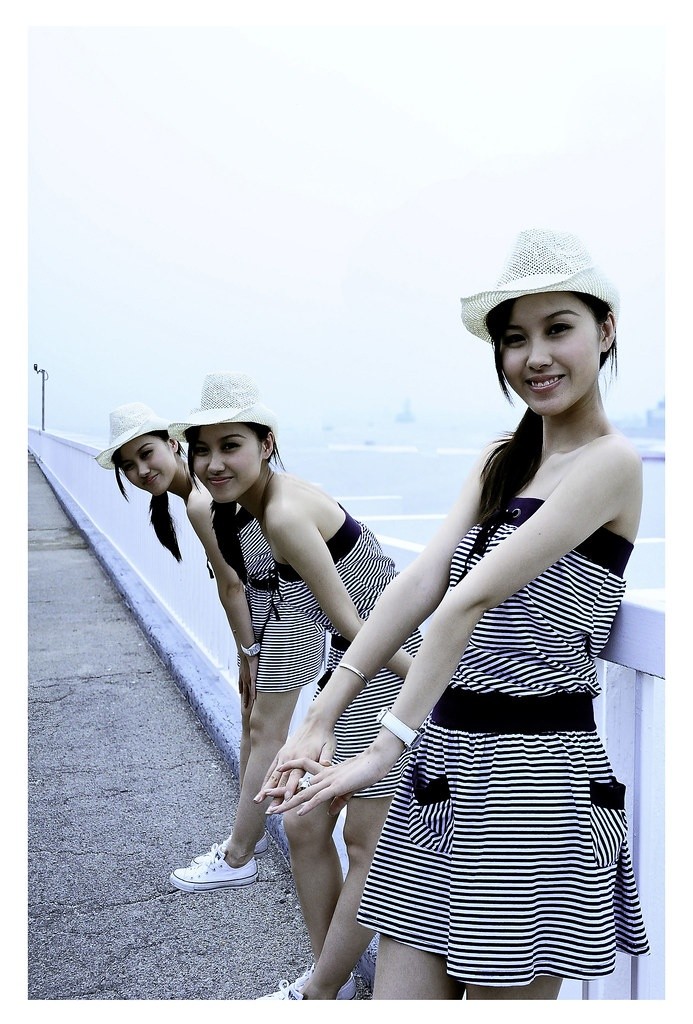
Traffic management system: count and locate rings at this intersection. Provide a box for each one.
[300,777,312,790]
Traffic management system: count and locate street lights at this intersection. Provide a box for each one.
[33,363,48,435]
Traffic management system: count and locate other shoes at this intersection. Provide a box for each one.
[293,964,356,1000]
[257,980,304,1001]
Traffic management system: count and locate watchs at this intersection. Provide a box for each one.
[240,643,261,656]
[376,707,425,750]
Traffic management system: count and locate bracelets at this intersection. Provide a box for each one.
[339,663,371,686]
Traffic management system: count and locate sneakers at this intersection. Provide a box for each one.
[191,831,268,866]
[170,846,259,892]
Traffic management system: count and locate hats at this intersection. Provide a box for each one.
[95,403,174,470]
[459,228,620,345]
[168,372,279,442]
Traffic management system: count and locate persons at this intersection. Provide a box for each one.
[96,400,326,893]
[166,370,423,1000]
[255,227,649,999]
